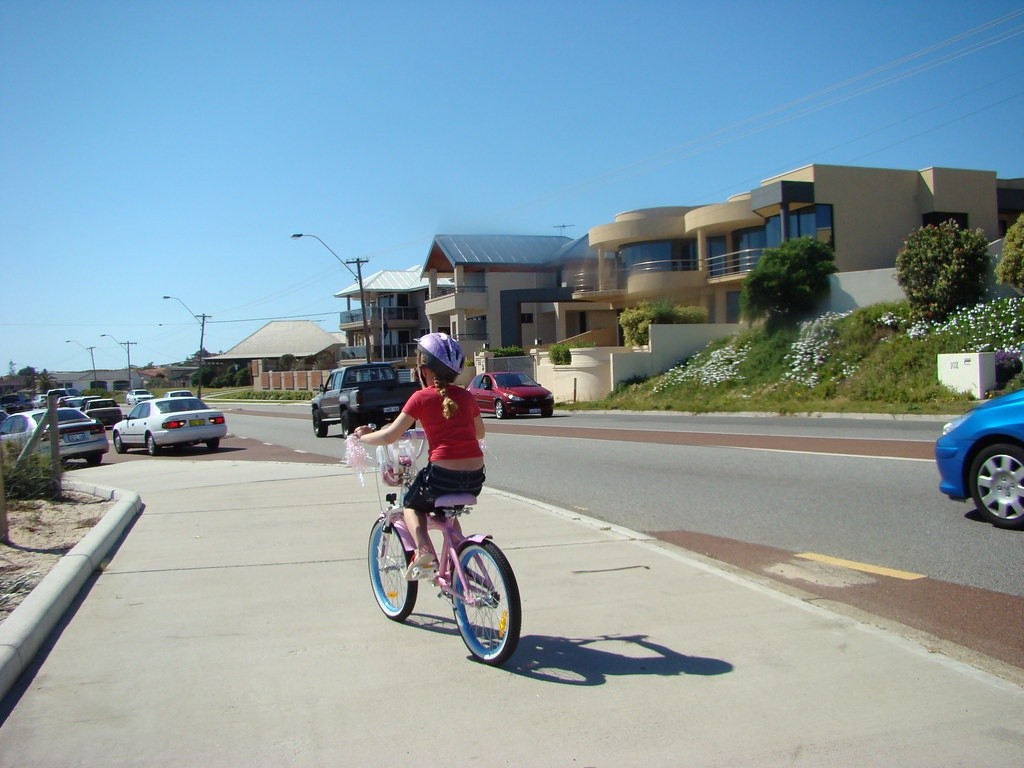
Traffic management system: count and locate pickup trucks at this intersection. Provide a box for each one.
[310,363,424,440]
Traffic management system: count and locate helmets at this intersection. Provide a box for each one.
[413,332,466,377]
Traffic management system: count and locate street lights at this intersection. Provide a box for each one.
[163,296,205,399]
[65,340,98,391]
[100,334,131,391]
[290,233,370,363]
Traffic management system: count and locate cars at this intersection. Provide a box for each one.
[0,407,109,467]
[0,388,122,430]
[463,370,556,421]
[126,389,155,406]
[161,389,195,398]
[112,397,228,456]
[933,388,1024,529]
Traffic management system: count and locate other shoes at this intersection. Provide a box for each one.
[406,544,436,582]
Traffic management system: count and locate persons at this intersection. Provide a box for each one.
[354,333,485,580]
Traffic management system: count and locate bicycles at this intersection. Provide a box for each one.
[347,422,523,665]
